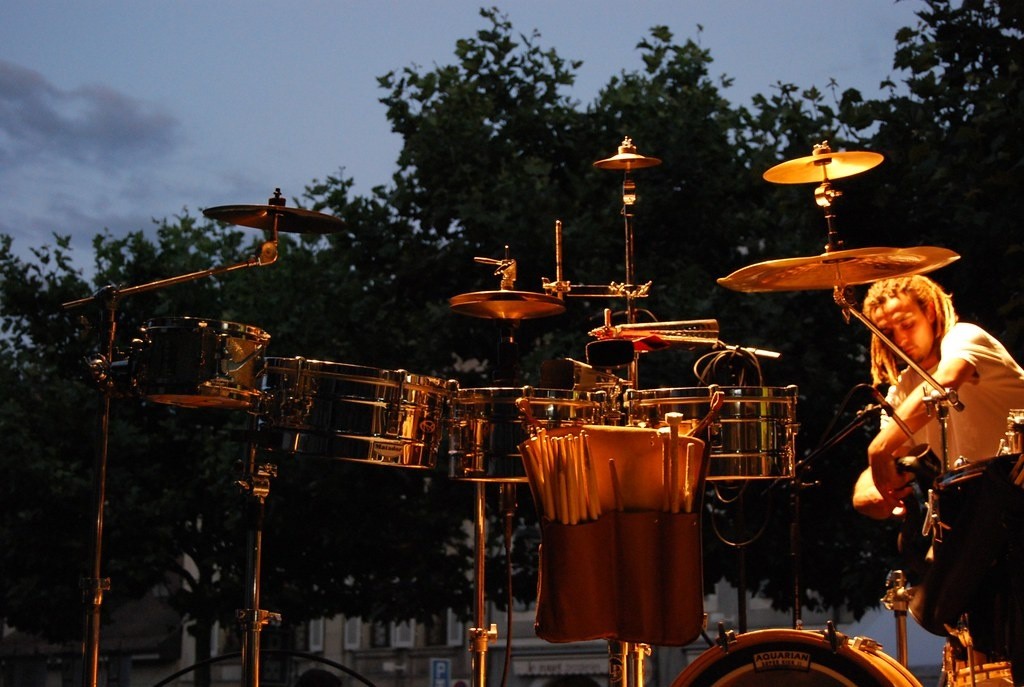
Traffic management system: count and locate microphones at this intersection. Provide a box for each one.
[868,384,914,440]
[724,345,781,359]
[590,308,639,324]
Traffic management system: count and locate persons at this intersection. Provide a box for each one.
[851,274,1024,636]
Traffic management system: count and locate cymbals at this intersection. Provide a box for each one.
[206,205,345,235]
[596,155,663,171]
[761,150,885,190]
[718,245,963,297]
[452,291,566,319]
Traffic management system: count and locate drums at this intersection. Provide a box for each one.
[1005,408,1024,457]
[237,350,449,469]
[668,625,924,687]
[136,311,264,405]
[440,381,618,483]
[625,384,799,478]
[896,453,1024,638]
